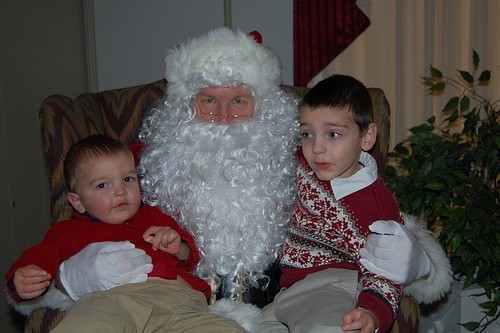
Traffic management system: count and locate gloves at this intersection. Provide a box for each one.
[60,240,154,302]
[359,220,431,285]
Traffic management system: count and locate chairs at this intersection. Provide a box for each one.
[25,78,421,333]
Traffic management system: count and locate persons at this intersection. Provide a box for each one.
[255,74,405,333]
[4,134,251,333]
[5,27,453,333]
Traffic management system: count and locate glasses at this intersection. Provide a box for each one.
[196,96,255,124]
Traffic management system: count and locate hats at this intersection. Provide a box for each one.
[164,27,281,100]
